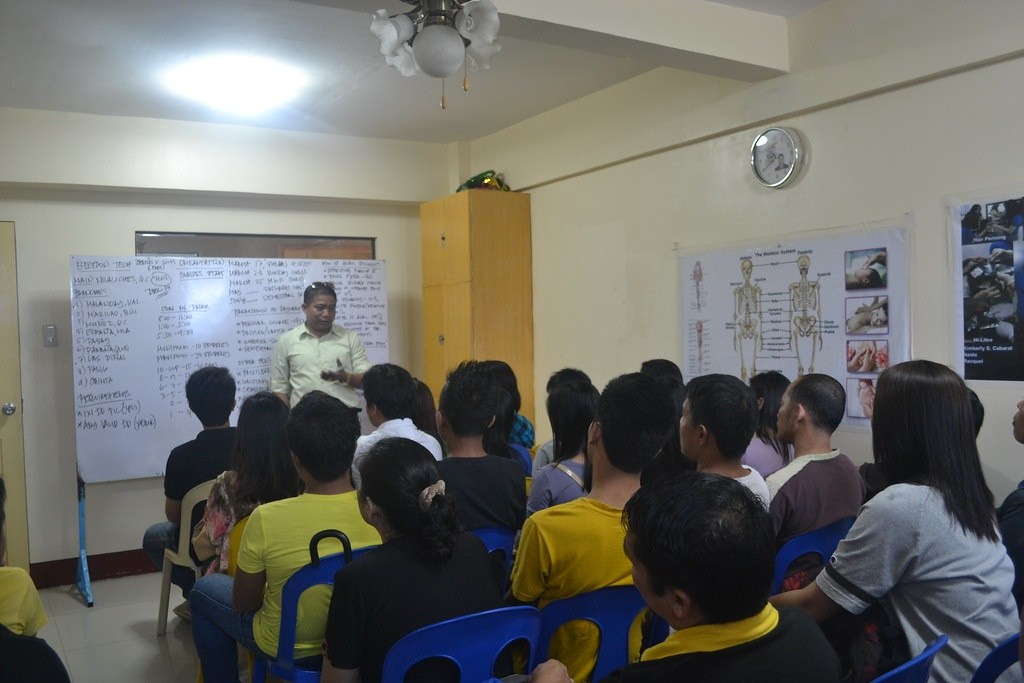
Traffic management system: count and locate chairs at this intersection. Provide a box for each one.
[255,545,380,683]
[871,634,949,683]
[540,585,670,683]
[157,480,216,636]
[195,516,255,683]
[970,630,1024,683]
[381,605,540,683]
[470,527,514,578]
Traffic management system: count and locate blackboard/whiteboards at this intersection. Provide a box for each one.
[70,256,388,483]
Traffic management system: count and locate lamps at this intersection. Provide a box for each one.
[370,0,499,110]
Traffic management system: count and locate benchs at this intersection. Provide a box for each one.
[772,517,855,594]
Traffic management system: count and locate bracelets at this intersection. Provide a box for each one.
[338,372,351,386]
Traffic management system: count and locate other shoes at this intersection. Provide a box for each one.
[174,600,193,622]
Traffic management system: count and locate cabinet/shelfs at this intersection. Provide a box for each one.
[420,189,534,433]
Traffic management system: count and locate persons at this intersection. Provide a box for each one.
[269,282,370,440]
[962,200,1024,344]
[0,477,71,683]
[845,254,889,373]
[144,360,1024,683]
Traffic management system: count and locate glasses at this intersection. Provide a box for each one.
[311,281,335,290]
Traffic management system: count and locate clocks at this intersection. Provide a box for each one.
[750,126,802,188]
